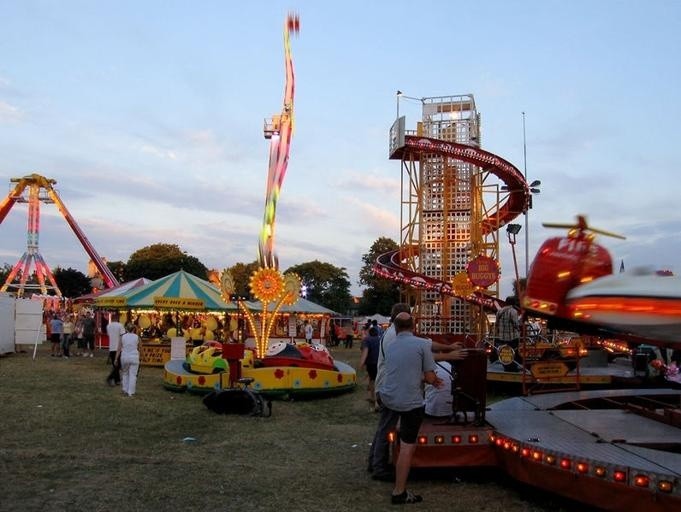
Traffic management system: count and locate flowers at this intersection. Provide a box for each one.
[218,267,302,359]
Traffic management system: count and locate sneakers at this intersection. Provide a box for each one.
[391,489,423,505]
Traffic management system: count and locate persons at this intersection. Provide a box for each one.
[386,312,434,503]
[304,320,314,344]
[346,324,355,348]
[47,305,97,360]
[114,324,143,399]
[367,303,470,480]
[526,317,543,335]
[331,319,340,346]
[106,314,126,387]
[495,296,520,373]
[423,352,457,420]
[361,318,386,404]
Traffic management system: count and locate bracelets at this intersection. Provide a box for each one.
[115,358,118,360]
